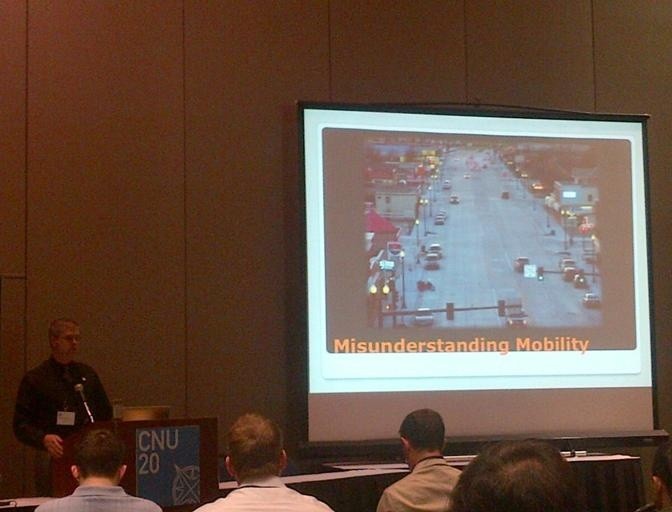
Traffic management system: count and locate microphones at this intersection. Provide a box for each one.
[73,382,96,425]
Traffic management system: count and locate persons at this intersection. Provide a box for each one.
[443,439,586,511]
[375,408,463,511]
[191,411,334,511]
[633,439,672,512]
[12,318,115,498]
[32,429,163,512]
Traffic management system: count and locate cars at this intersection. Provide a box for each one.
[415,306,434,326]
[502,308,529,328]
[558,258,601,308]
[433,207,449,225]
[422,241,443,271]
[440,150,509,190]
[447,194,460,204]
[512,256,532,275]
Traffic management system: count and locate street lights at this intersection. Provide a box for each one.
[506,158,527,202]
[414,217,420,265]
[368,281,392,331]
[419,198,430,238]
[425,163,443,216]
[398,249,407,312]
[560,208,573,252]
[591,232,596,283]
[532,183,544,214]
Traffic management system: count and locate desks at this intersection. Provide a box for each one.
[0,496,56,512]
[219,451,647,512]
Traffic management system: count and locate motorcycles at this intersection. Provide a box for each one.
[417,280,438,291]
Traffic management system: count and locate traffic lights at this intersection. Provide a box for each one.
[537,267,545,281]
